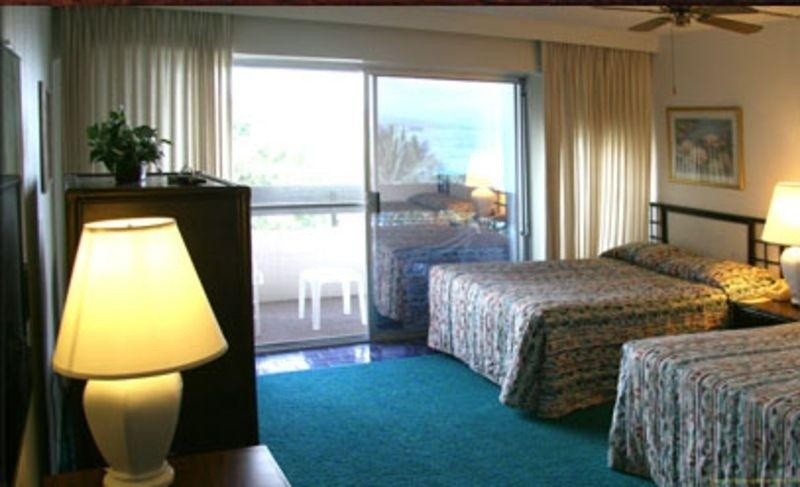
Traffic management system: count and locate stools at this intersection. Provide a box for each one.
[295,265,369,331]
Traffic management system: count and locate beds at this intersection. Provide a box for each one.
[607,320,800,487]
[428,204,769,420]
[371,193,512,328]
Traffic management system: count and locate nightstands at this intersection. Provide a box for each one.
[725,291,799,331]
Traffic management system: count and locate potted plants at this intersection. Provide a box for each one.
[86,103,173,184]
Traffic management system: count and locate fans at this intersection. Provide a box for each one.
[603,0,763,37]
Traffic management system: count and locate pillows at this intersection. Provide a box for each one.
[597,242,687,266]
[657,254,775,301]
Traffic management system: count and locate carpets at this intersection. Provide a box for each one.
[255,354,660,486]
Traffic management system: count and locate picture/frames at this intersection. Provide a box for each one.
[665,107,745,190]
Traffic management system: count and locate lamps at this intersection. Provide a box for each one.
[50,218,229,486]
[761,182,800,304]
